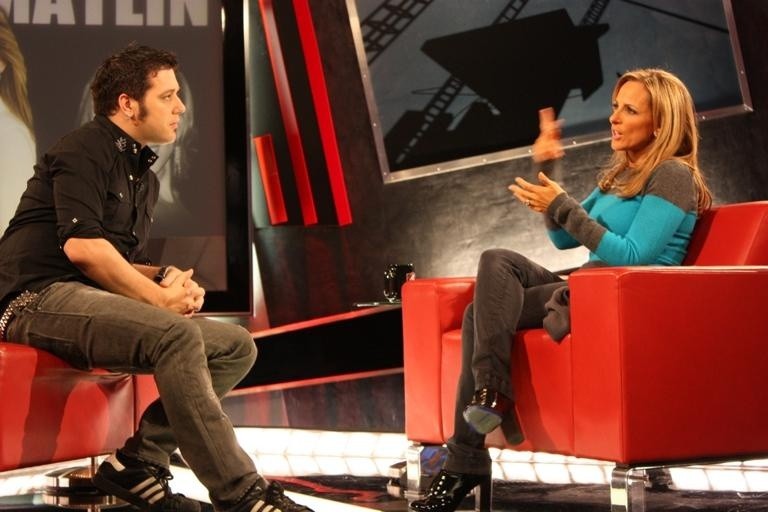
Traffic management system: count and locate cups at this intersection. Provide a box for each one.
[385,262,415,304]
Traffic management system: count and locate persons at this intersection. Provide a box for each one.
[407,67,713,511]
[77,58,198,237]
[0,40,316,512]
[0,10,38,240]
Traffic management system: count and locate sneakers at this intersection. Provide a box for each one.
[208,475,317,512]
[91,443,204,512]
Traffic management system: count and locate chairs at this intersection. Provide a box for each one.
[399,199,768,511]
[0,337,160,506]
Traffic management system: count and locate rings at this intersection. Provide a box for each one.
[525,200,530,206]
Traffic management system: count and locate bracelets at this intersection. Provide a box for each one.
[152,266,167,285]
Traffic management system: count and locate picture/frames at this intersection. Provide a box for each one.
[344,1,751,186]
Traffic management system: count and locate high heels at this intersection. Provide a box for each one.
[408,468,495,512]
[461,384,526,450]
